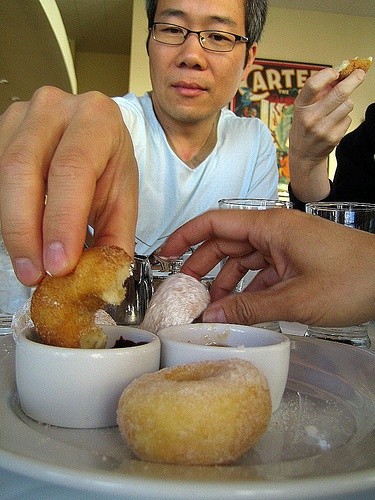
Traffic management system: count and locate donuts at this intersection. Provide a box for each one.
[116,357,272,465]
[340,54,373,79]
[29,246,134,348]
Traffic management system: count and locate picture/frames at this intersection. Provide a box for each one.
[229,58,333,199]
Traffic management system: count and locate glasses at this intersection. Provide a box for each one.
[150,21,253,52]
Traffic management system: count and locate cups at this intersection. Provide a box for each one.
[220,199,294,295]
[305,201,374,348]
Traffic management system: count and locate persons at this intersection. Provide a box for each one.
[0,0,277,294]
[154,208,375,327]
[289,67,374,234]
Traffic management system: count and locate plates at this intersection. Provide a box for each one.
[1,335,375,498]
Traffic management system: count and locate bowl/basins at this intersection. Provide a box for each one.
[13,325,160,430]
[158,322,291,414]
